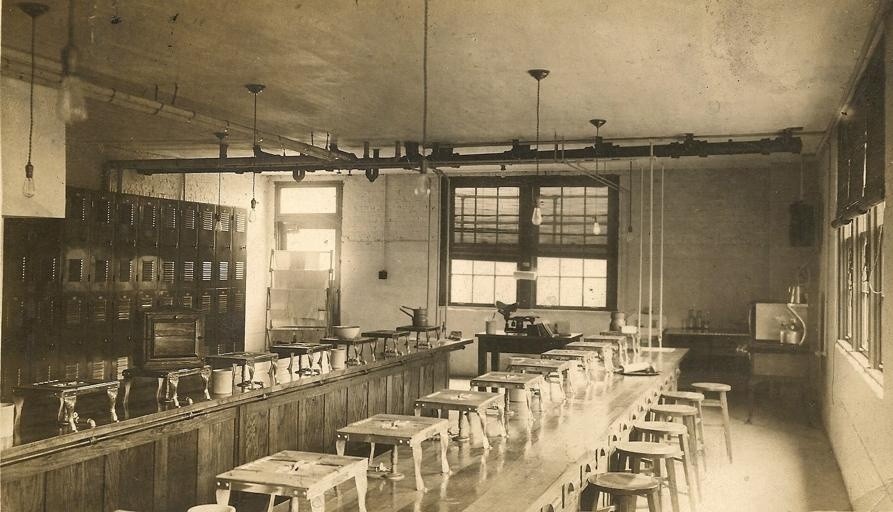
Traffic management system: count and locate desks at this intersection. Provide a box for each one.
[8,325,640,512]
[663,328,816,428]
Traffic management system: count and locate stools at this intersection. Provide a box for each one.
[580,382,732,512]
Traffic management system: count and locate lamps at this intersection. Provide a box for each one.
[18,1,87,197]
[213,83,266,232]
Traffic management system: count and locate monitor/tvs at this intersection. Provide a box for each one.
[143,311,204,371]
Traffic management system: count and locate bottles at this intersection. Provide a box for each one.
[778,317,801,345]
[680,306,712,331]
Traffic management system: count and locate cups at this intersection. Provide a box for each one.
[329,348,347,372]
[0,402,15,439]
[211,368,233,395]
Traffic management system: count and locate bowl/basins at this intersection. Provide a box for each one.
[332,324,361,341]
